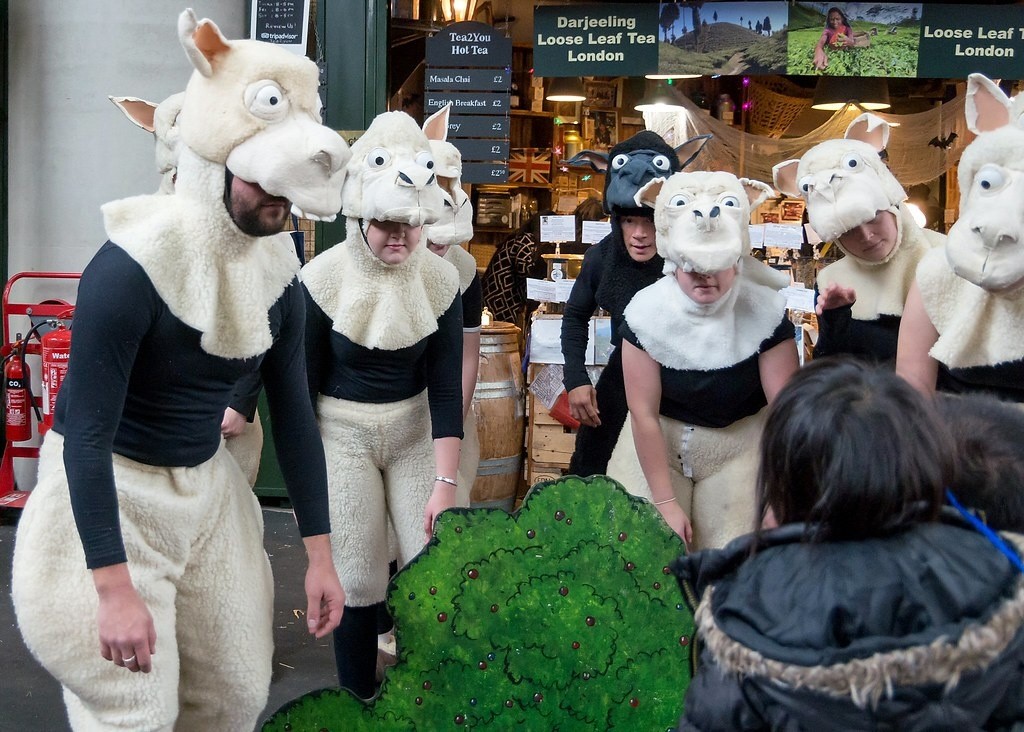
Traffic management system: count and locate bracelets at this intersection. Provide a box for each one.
[434,476,458,486]
[654,496,677,506]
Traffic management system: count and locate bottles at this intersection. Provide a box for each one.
[562,129,581,161]
[521,200,538,223]
[511,84,520,109]
[716,94,735,125]
[695,92,711,115]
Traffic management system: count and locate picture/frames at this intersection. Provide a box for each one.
[581,104,623,149]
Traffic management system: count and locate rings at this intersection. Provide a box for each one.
[121,655,136,664]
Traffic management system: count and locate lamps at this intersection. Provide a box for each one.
[810,75,891,111]
[546,77,586,102]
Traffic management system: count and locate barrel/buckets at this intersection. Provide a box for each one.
[540,253,585,314]
[469,318,527,514]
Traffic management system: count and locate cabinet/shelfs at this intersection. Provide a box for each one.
[387,18,555,276]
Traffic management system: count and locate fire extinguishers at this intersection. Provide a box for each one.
[0,333,37,441]
[21,308,76,434]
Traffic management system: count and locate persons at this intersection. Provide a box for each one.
[769,112,949,360]
[11,7,350,732]
[594,122,611,146]
[869,27,877,36]
[560,195,606,254]
[561,130,714,478]
[892,72,1024,402]
[812,6,854,71]
[476,209,559,325]
[890,26,897,34]
[291,110,464,703]
[605,172,800,557]
[405,102,481,506]
[672,353,1024,732]
